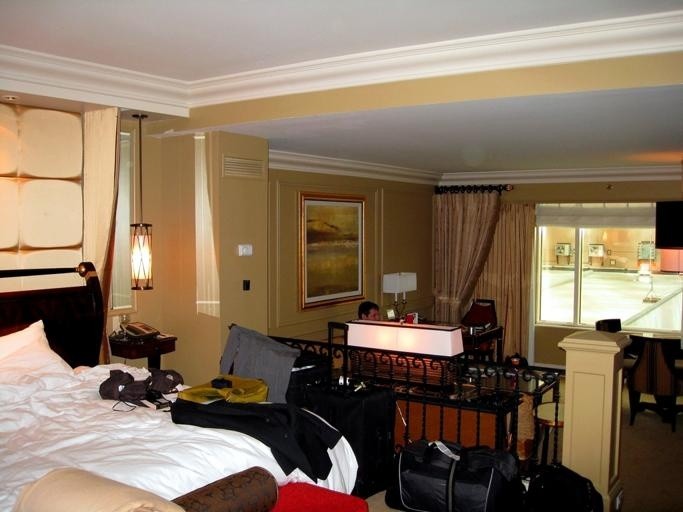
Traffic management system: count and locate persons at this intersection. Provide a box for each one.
[358,302,380,320]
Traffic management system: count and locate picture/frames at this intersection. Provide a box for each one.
[297,192,368,311]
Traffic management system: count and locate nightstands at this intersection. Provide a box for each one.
[109,332,178,371]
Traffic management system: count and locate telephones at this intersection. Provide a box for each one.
[120,321,161,339]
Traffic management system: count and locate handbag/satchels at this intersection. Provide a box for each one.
[385,439,522,512]
[497,464,604,512]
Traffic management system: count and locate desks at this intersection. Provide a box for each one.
[428,322,504,364]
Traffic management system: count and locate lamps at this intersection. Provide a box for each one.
[381,272,418,318]
[130,113,152,292]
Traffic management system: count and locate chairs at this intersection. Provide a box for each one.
[461,297,497,362]
[328,321,349,345]
[625,341,683,432]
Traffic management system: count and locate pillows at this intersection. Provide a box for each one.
[0,323,74,379]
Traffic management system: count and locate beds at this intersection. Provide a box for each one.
[0,262,359,512]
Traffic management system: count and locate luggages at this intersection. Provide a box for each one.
[317,323,395,500]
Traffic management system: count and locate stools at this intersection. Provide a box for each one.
[272,483,370,511]
